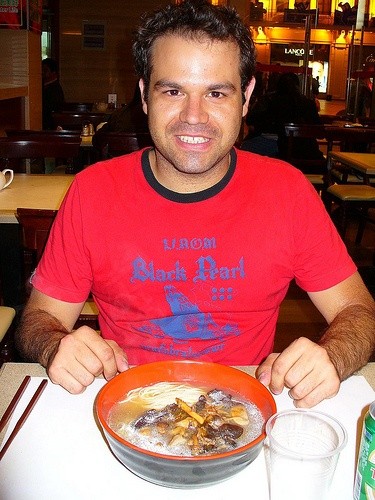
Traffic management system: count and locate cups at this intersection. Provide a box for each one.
[265,408,348,500]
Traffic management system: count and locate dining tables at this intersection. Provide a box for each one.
[0,362,375,500]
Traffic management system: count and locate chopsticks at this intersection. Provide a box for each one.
[0,375,47,460]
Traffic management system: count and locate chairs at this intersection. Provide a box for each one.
[0,102,375,352]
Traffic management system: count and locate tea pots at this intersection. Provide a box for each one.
[95,102,108,112]
[0,169,14,190]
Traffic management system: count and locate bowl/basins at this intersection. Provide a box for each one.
[93,359,277,491]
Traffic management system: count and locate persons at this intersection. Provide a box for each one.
[253,72,325,160]
[41,58,67,129]
[16,0,374,410]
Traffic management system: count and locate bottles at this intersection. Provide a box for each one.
[82,119,94,135]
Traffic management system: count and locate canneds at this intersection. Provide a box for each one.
[353,401,375,500]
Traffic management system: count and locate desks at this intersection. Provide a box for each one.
[0,174,74,247]
[80,135,95,165]
[333,153,375,184]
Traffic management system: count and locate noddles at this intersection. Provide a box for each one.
[117,381,207,409]
